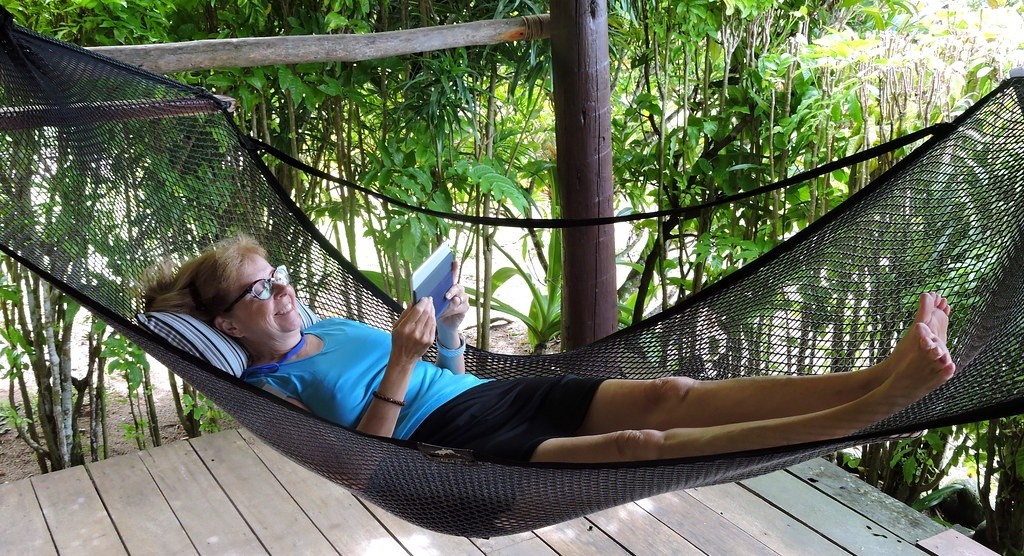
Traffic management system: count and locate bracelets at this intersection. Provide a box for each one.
[372,391,406,407]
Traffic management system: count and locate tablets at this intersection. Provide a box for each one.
[410,241,454,319]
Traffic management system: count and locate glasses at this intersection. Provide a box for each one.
[224,264,290,314]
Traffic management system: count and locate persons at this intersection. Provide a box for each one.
[139,231,956,537]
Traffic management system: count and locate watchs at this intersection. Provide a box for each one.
[436,332,466,358]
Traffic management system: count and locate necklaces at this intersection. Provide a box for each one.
[242,329,306,378]
